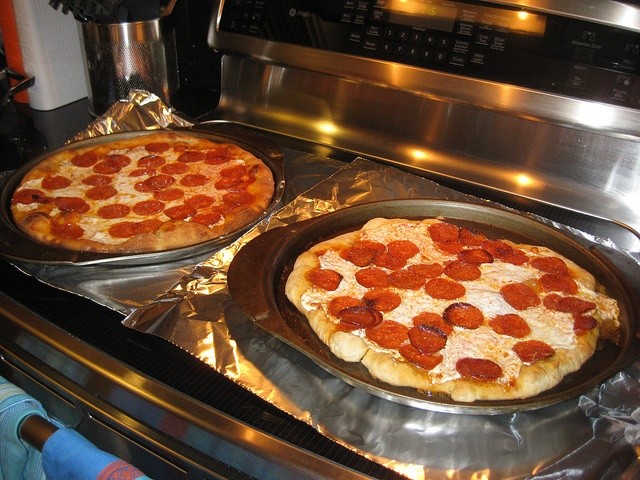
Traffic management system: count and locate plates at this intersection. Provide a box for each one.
[227,197,639,415]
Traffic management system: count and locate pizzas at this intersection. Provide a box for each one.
[282,215,623,404]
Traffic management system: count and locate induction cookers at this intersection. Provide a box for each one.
[0,111,639,478]
